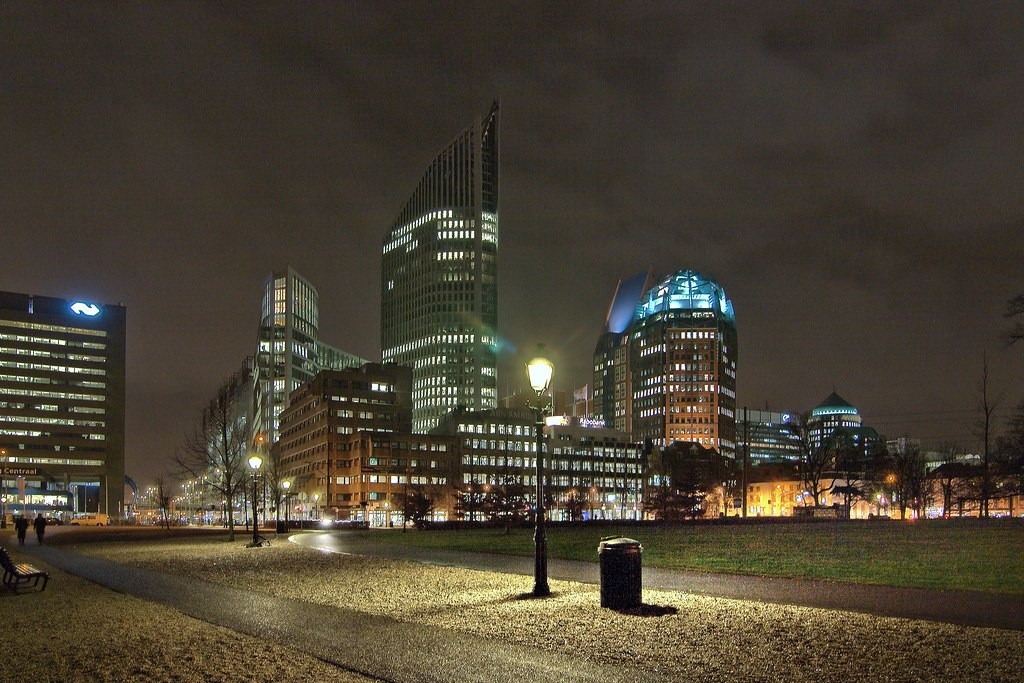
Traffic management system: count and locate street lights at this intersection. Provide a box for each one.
[248,456,263,545]
[283,480,291,533]
[523,342,556,596]
[384,502,389,527]
[197,474,207,526]
[1,496,7,528]
[222,499,226,528]
[313,492,320,519]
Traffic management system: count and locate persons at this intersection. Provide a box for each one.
[197,517,252,528]
[34,513,47,546]
[581,515,584,521]
[545,517,550,522]
[15,514,28,545]
[595,515,597,520]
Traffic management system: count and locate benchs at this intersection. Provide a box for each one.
[0,546,49,593]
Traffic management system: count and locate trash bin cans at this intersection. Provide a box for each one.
[597,537,642,611]
[277,520,285,534]
[1,520,6,529]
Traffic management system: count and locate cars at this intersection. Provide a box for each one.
[48,518,63,525]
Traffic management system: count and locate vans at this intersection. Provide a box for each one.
[71,514,110,526]
